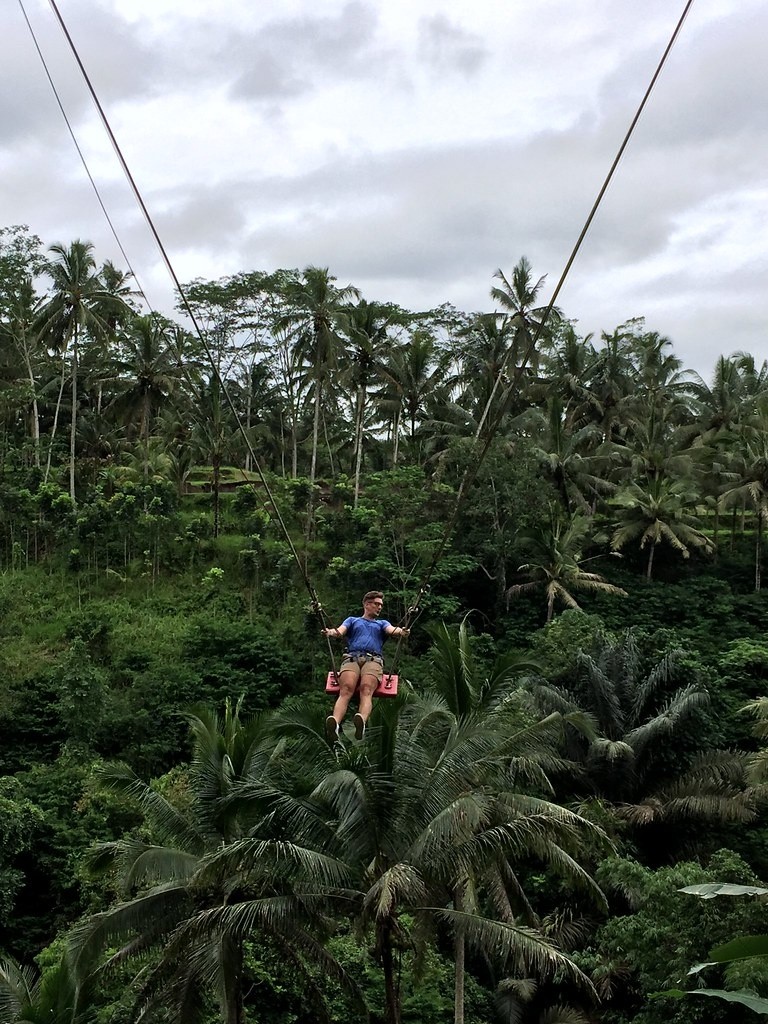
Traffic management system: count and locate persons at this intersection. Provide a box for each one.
[321,592,410,741]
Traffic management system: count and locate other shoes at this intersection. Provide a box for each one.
[326,716,340,743]
[354,711,366,740]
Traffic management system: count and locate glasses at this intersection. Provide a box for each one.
[367,602,384,608]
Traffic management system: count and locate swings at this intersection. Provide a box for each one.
[48,1,696,699]
